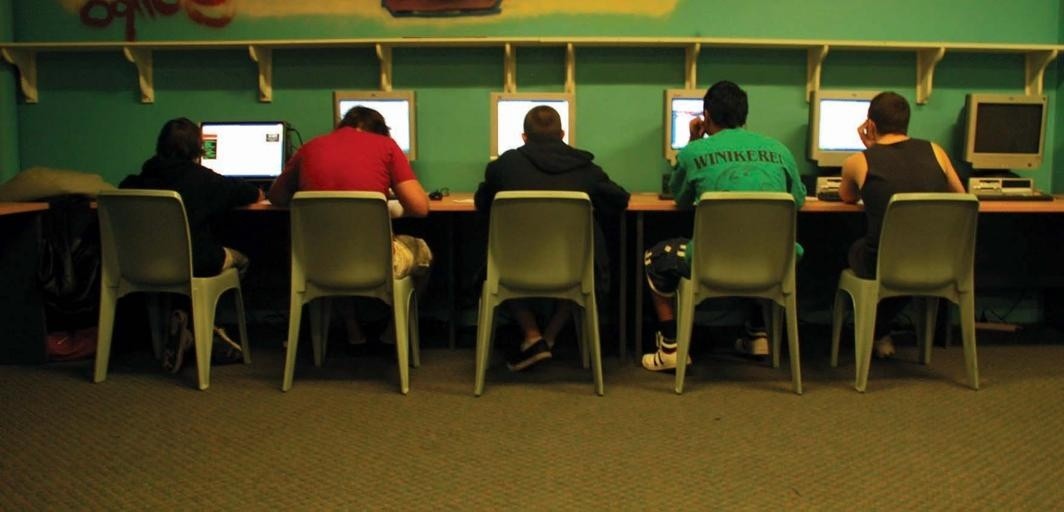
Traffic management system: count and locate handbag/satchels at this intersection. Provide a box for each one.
[39,323,103,363]
[25,194,109,316]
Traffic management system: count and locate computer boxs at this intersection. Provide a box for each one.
[958,166,1035,197]
[801,173,846,196]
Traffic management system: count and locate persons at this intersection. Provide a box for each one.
[269,107,434,356]
[119,117,264,364]
[642,81,807,371]
[840,92,966,358]
[475,106,631,372]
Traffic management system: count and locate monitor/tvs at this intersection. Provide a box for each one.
[807,90,895,176]
[333,88,417,162]
[953,92,1052,178]
[665,89,728,167]
[488,92,578,160]
[195,119,288,183]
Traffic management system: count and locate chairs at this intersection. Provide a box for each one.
[468,189,610,401]
[669,189,806,396]
[825,189,982,401]
[89,187,255,393]
[280,189,428,395]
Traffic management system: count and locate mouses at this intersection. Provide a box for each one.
[428,190,444,201]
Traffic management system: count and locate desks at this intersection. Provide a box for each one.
[81,180,1064,370]
[0,197,59,368]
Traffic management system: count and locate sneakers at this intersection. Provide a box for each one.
[212,323,243,361]
[735,329,772,356]
[640,341,692,372]
[506,339,551,371]
[159,310,189,376]
[875,336,898,359]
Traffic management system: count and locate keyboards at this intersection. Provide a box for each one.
[817,191,840,202]
[979,193,1055,201]
